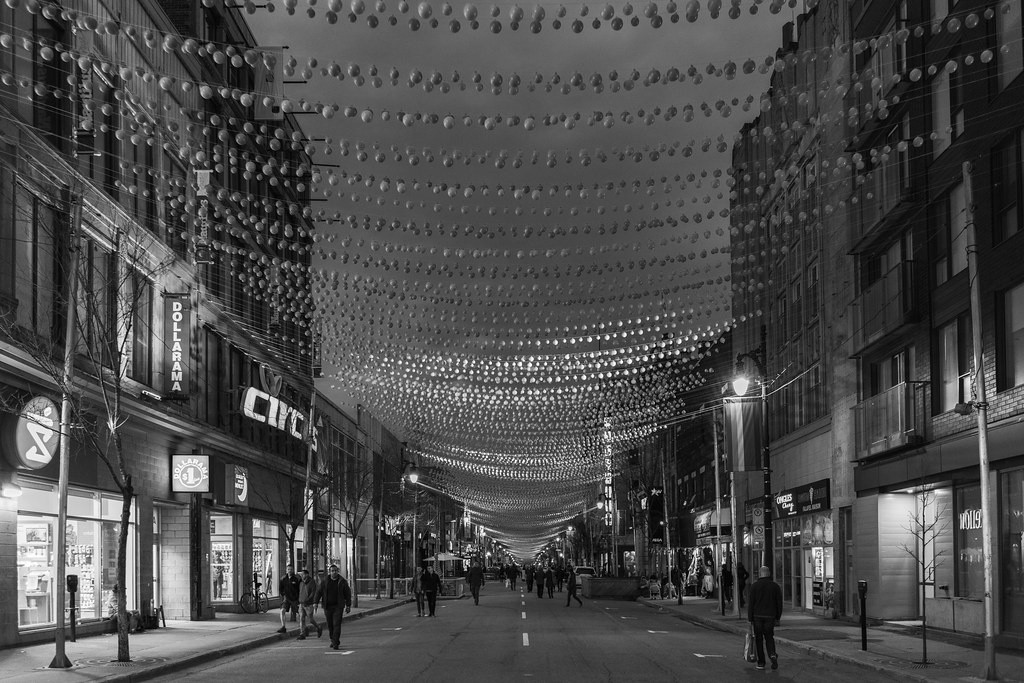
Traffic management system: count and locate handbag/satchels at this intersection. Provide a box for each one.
[743,621,757,663]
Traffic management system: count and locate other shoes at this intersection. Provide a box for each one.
[316,625,322,637]
[330,639,333,648]
[756,664,765,669]
[333,646,339,650]
[429,612,435,617]
[277,628,287,633]
[770,655,778,670]
[296,635,305,640]
[416,613,425,617]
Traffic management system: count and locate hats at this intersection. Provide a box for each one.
[759,566,770,578]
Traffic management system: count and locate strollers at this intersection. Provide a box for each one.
[649,580,662,600]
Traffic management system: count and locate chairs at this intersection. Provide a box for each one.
[649,585,661,599]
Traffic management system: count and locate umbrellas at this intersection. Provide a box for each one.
[423,553,464,582]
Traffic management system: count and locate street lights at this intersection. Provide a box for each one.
[399,447,419,594]
[458,509,469,571]
[597,485,612,577]
[734,324,773,579]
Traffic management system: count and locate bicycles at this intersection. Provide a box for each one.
[240,581,269,614]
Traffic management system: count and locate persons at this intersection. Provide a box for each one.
[747,565,783,669]
[554,566,565,592]
[296,570,323,639]
[313,564,351,649]
[526,566,533,592]
[565,568,582,606]
[410,565,425,617]
[213,552,224,600]
[567,562,573,567]
[717,564,733,604]
[671,564,685,596]
[277,564,299,632]
[543,567,554,599]
[737,562,749,608]
[499,563,520,591]
[421,565,442,617]
[467,562,484,604]
[534,568,546,598]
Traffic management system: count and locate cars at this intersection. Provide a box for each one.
[486,567,507,581]
[573,566,597,586]
